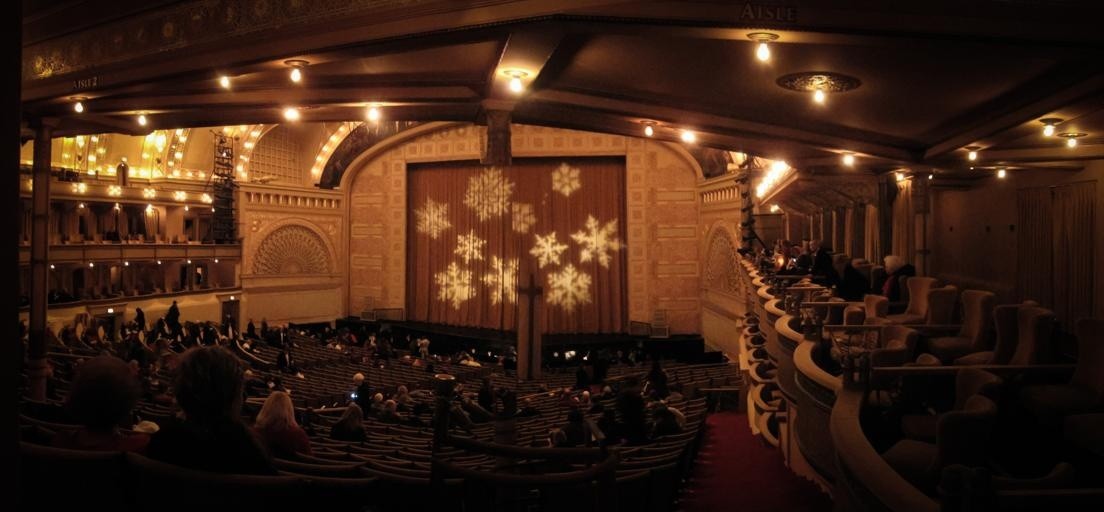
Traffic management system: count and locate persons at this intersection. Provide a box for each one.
[871,254,906,303]
[331,371,547,447]
[541,343,687,471]
[19,299,294,404]
[140,348,277,474]
[55,287,73,302]
[20,354,165,475]
[291,321,518,371]
[256,390,312,455]
[741,235,842,287]
[106,223,118,240]
[48,288,57,305]
[834,252,865,303]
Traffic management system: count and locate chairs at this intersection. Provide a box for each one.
[771,252,1104,487]
[0,330,747,512]
